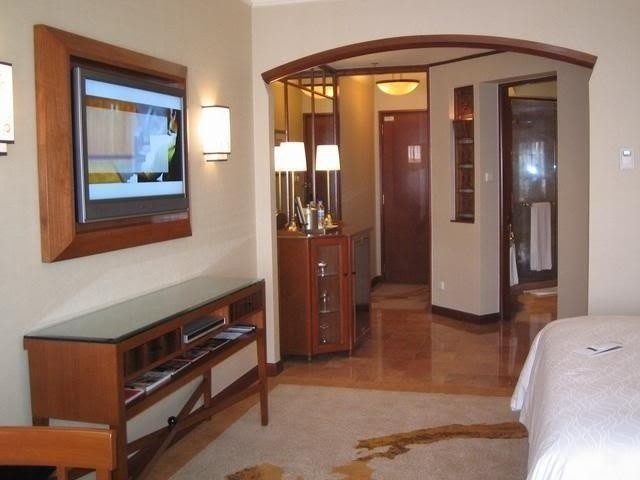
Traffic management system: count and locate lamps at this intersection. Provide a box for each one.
[314,145,344,229]
[274,140,308,232]
[376,79,420,97]
[0,60,16,156]
[200,104,232,163]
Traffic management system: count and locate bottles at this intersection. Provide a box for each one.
[303,201,325,230]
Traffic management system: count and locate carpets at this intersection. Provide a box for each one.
[165,381,529,478]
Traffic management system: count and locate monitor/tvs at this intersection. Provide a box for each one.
[73,66,188,224]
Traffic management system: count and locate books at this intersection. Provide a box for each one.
[124,323,257,404]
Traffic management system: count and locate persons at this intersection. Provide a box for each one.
[135,108,182,182]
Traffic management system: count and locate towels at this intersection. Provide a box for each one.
[529,200,552,270]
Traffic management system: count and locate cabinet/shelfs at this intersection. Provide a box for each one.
[278,220,375,362]
[21,275,268,479]
[448,83,476,223]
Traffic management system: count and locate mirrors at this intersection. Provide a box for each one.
[273,63,343,236]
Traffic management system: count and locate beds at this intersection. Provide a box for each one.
[507,313,639,477]
